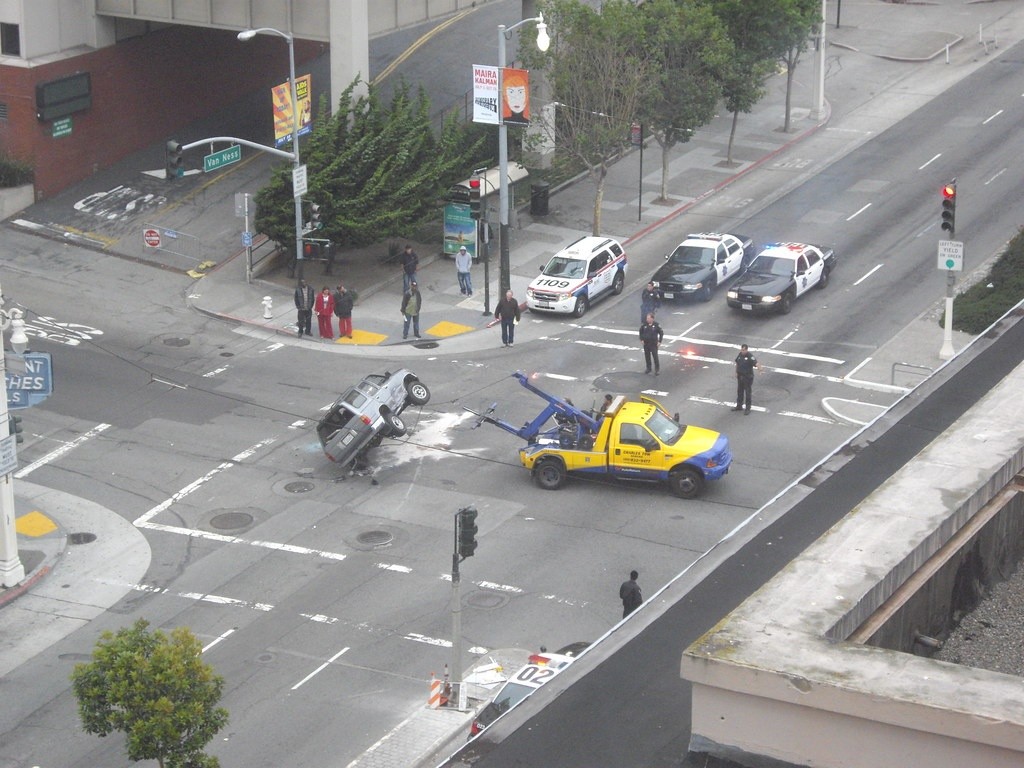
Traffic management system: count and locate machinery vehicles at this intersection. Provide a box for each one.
[461,370,732,499]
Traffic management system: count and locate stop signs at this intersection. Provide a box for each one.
[143,228,161,248]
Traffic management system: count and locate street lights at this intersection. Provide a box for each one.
[497,10,550,320]
[236,27,306,291]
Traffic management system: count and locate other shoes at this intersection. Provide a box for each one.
[646,369,651,373]
[403,334,407,339]
[501,344,507,347]
[656,370,660,375]
[298,331,352,341]
[732,406,742,411]
[744,408,750,414]
[415,333,421,338]
[509,343,513,346]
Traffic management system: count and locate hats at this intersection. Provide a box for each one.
[405,244,411,248]
[411,281,417,285]
[460,246,466,250]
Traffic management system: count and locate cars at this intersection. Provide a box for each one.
[725,238,837,315]
[468,641,593,741]
[317,367,430,469]
[650,231,756,302]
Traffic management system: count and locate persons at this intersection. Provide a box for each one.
[333,285,353,339]
[495,290,520,348]
[400,281,421,339]
[639,314,664,376]
[730,344,762,415]
[400,244,419,292]
[314,287,335,341]
[455,246,473,298]
[294,277,315,338]
[640,282,661,323]
[620,570,643,619]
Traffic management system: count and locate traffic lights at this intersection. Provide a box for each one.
[468,175,482,220]
[308,202,323,231]
[942,183,955,235]
[303,241,335,262]
[165,139,184,184]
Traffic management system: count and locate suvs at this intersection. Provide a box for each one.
[525,234,629,315]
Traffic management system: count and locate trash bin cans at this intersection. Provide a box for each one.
[529,180,549,216]
